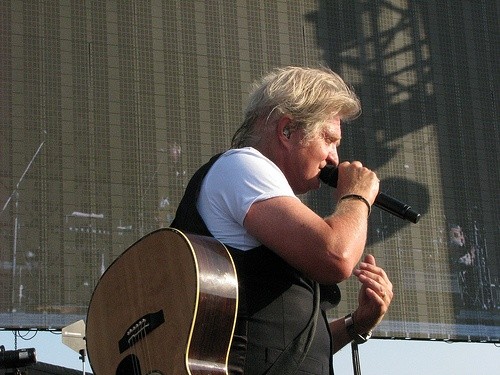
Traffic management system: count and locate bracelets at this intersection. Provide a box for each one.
[337,194,371,216]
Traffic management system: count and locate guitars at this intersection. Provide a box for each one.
[86,228,241,375]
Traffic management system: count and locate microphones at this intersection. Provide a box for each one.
[319,163,422,225]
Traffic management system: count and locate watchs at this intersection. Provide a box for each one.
[343,312,372,345]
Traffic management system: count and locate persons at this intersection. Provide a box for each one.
[169,64,394,375]
